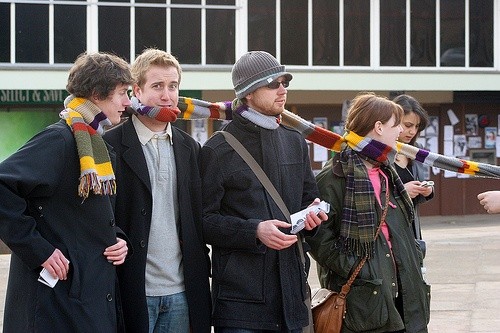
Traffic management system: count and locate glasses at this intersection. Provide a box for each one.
[263,80,289,88]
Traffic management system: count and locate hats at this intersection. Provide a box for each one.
[232,50,292,100]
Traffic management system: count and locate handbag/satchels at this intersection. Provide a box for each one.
[310,288,346,333]
[416,240,426,259]
[302,282,315,333]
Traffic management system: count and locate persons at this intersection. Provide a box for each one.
[102,47,213,333]
[488,132,495,141]
[392,94,436,241]
[0,53,135,333]
[306,93,431,333]
[316,121,325,129]
[202,51,329,333]
[477,190,499,214]
